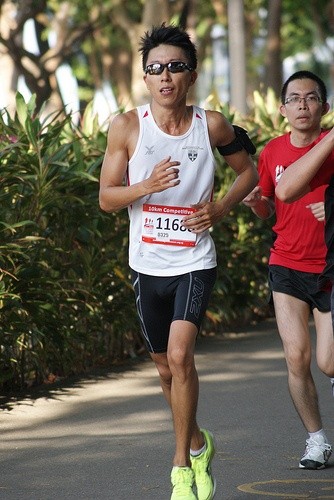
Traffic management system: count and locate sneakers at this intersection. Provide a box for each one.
[190,428,217,500]
[298,435,332,470]
[170,465,197,500]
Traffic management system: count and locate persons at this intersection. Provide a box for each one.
[275,125,334,337]
[100,23,259,500]
[241,70,334,471]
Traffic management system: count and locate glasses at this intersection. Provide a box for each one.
[283,96,325,105]
[144,62,193,75]
